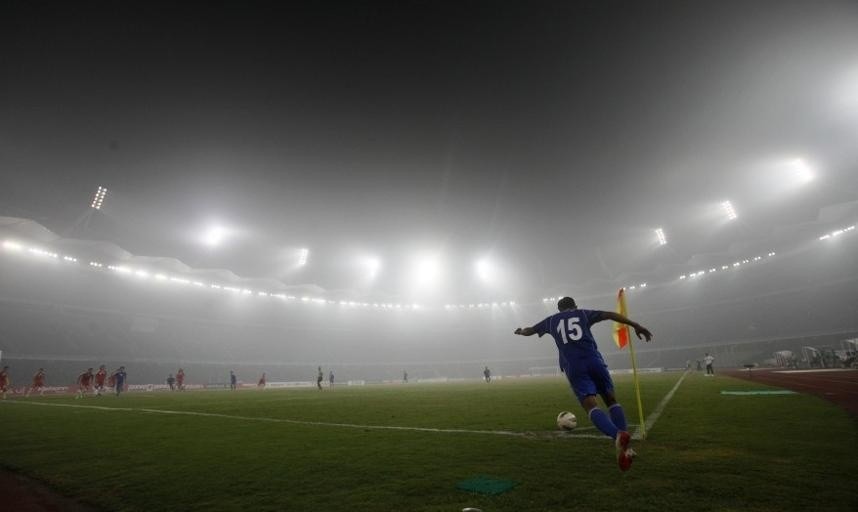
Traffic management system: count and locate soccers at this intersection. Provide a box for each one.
[557,411,577,431]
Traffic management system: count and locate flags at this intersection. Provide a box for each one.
[612,287,628,349]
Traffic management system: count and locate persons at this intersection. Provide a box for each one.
[695,357,703,372]
[230,370,237,390]
[686,358,693,372]
[76,364,128,399]
[26,367,48,398]
[166,374,175,391]
[785,349,858,370]
[402,370,409,384]
[329,371,335,392]
[704,352,715,376]
[317,366,323,391]
[254,372,269,392]
[176,368,186,391]
[0,365,10,400]
[514,296,654,471]
[484,366,492,383]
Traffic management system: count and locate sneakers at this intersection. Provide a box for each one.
[616,431,637,472]
